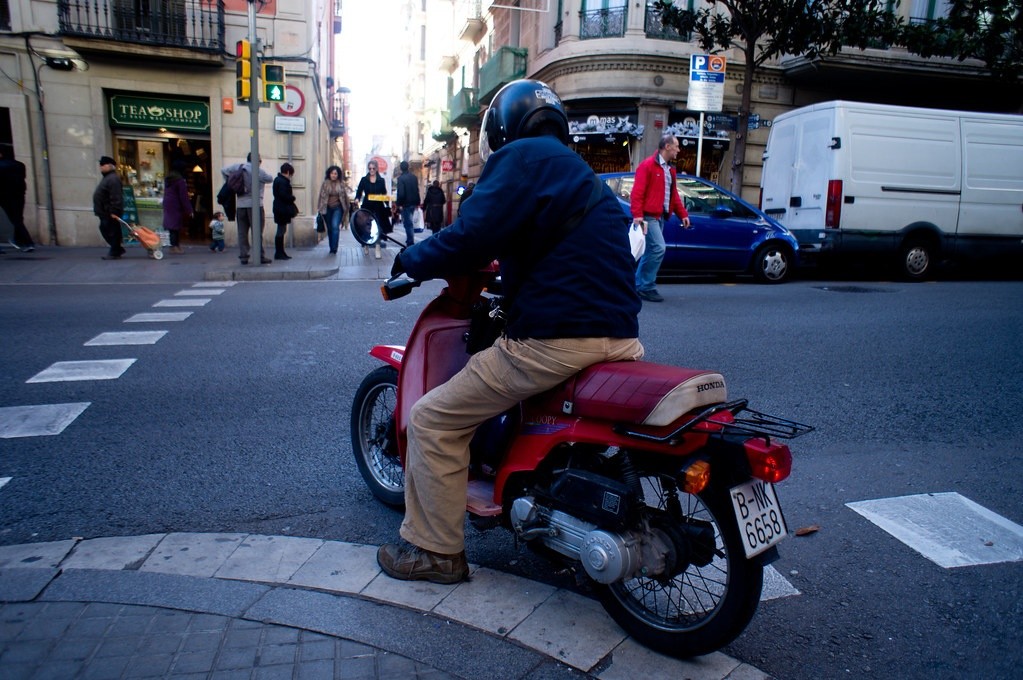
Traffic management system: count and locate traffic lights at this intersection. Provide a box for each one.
[235,40,251,99]
[261,64,287,104]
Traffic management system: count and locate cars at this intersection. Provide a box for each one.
[591,171,802,287]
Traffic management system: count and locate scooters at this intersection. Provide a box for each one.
[349,210,820,657]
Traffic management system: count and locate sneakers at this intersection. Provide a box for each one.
[378,537,468,584]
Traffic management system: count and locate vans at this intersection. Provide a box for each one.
[759,98,1023,284]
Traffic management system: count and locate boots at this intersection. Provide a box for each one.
[375,245,382,258]
[365,244,370,255]
[274,236,293,260]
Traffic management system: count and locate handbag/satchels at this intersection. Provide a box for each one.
[411,207,425,232]
[228,165,244,196]
[316,212,325,233]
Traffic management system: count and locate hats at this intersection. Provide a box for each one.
[99,156,116,166]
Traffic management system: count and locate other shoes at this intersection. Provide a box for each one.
[103,250,122,261]
[260,257,271,263]
[23,246,36,252]
[330,249,337,255]
[241,259,248,265]
[208,248,216,253]
[169,246,185,254]
[639,288,665,303]
[8,239,21,250]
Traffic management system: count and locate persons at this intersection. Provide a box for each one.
[209,212,226,254]
[375,77,645,584]
[272,163,296,261]
[92,156,127,261]
[421,180,446,232]
[221,151,274,265]
[163,166,194,256]
[352,160,392,259]
[317,165,348,255]
[0,145,36,252]
[628,134,690,304]
[395,161,420,248]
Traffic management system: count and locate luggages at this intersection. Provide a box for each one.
[110,212,163,261]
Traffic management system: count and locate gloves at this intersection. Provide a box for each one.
[629,220,648,261]
[390,250,404,277]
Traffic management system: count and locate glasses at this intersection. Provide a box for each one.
[368,168,376,171]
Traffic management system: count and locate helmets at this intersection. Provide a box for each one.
[485,79,568,154]
